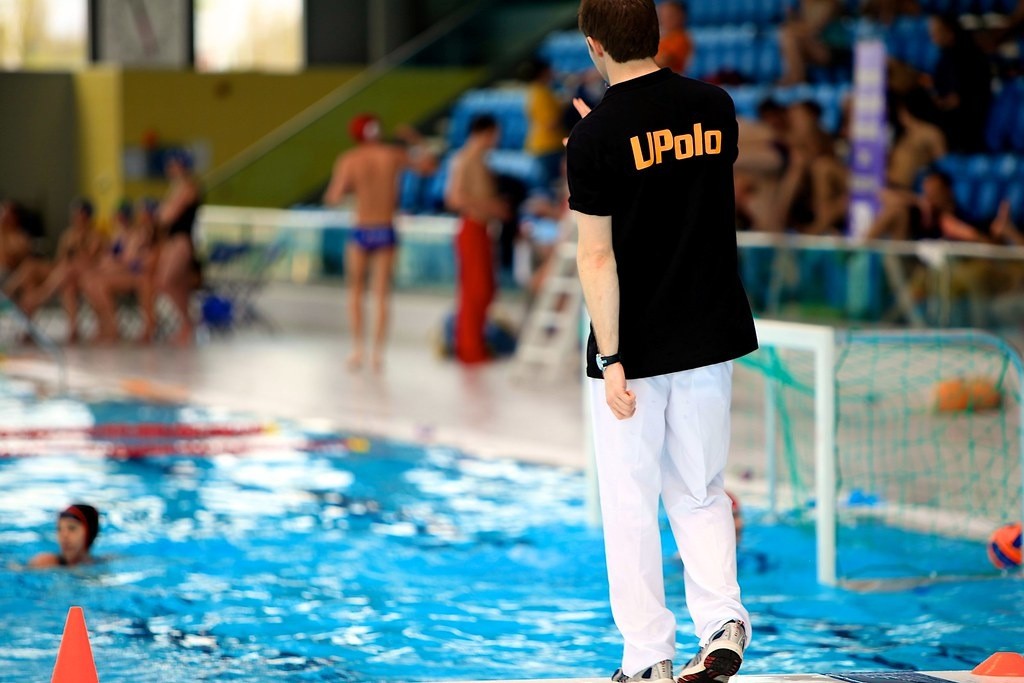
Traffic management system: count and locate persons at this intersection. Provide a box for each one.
[324,113,433,372]
[525,60,562,182]
[733,1,1024,321]
[573,0,694,99]
[445,112,507,363]
[564,1,759,683]
[0,142,204,346]
[33,503,99,565]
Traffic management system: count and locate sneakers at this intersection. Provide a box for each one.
[676,619,746,683]
[610,660,675,683]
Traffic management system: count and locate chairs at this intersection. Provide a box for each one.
[0,0,1024,349]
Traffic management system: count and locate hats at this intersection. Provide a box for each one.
[349,114,382,145]
[60,504,99,553]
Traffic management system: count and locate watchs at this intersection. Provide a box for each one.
[596,354,621,371]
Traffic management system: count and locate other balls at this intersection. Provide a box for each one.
[935,372,1006,410]
[987,522,1024,569]
